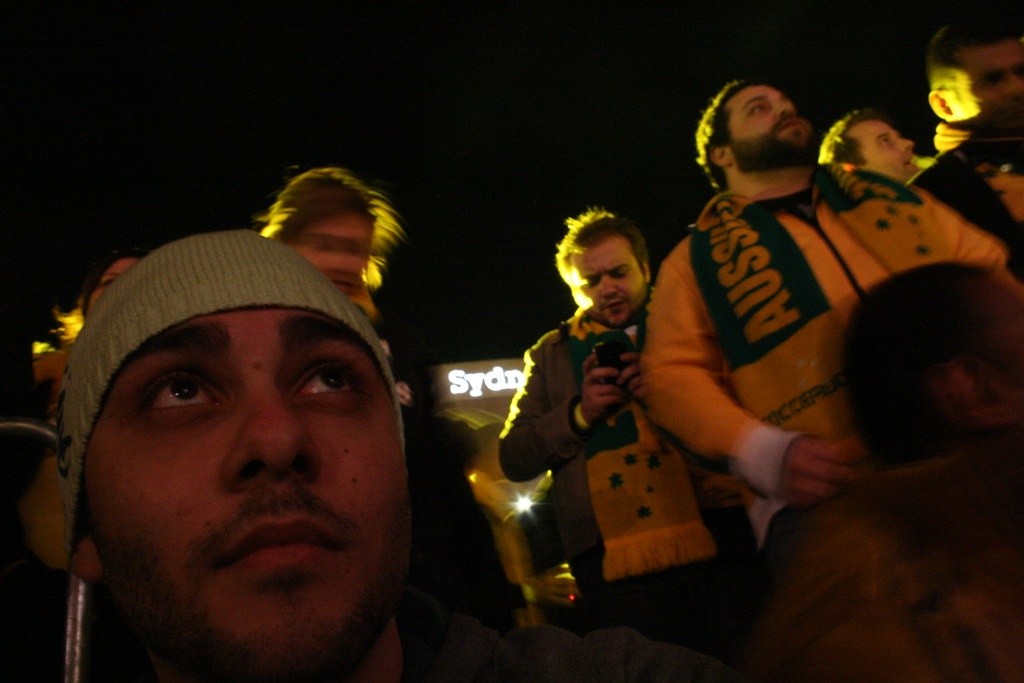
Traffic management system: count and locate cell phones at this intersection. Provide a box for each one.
[594,337,635,391]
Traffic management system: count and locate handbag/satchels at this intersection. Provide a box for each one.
[585,440,717,581]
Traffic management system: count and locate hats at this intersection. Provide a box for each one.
[55,228,409,562]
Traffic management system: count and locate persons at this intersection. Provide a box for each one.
[0,246,147,683]
[906,17,1024,278]
[740,260,1024,683]
[642,79,1024,548]
[57,230,741,683]
[261,167,405,319]
[496,208,759,658]
[818,110,925,185]
